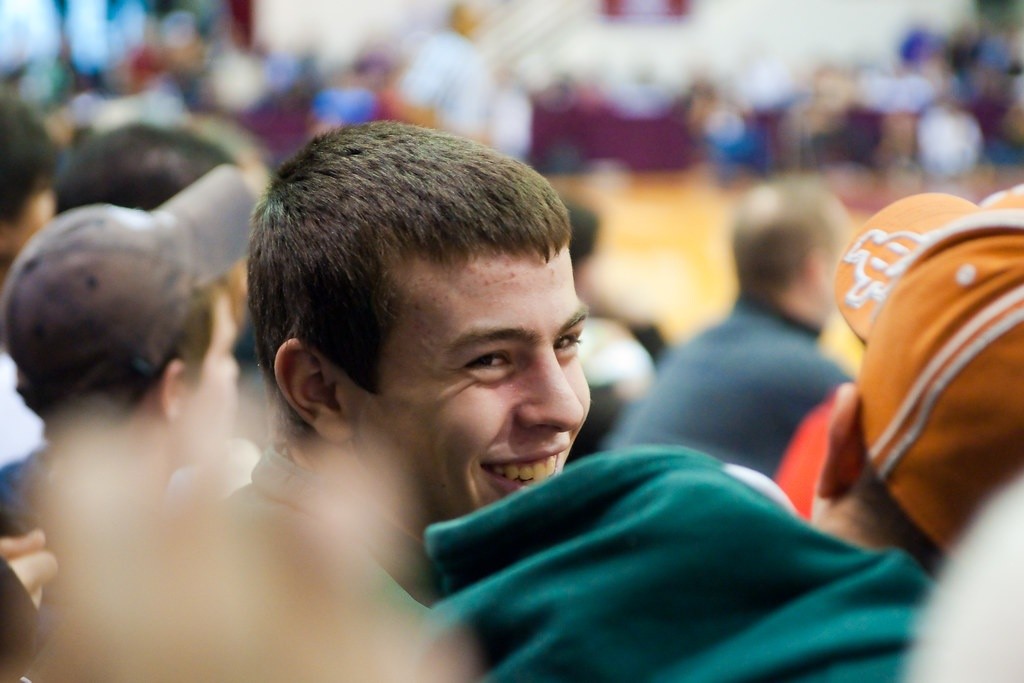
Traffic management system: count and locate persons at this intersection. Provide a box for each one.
[609,176,857,480]
[0,0,1024,683]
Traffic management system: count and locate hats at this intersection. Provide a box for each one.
[856,214,1024,547]
[980,184,1024,210]
[834,191,987,345]
[1,163,260,392]
